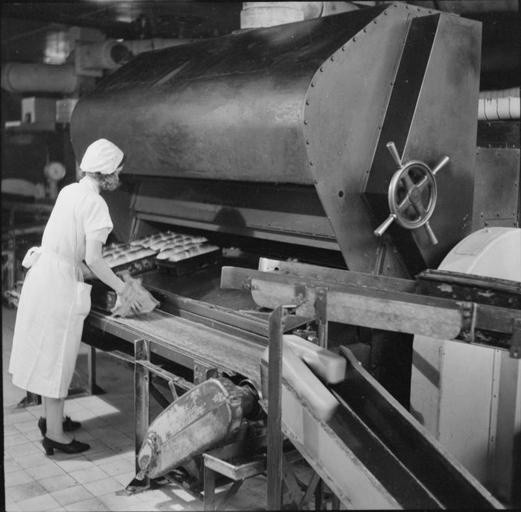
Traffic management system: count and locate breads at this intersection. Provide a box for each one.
[103,230,218,268]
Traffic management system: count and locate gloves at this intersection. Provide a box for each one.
[117,283,145,312]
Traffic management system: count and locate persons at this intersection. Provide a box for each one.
[6,135,151,456]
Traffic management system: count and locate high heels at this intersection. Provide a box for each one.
[43,437,90,455]
[39,414,82,437]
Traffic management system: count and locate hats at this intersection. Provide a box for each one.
[80,139,126,174]
